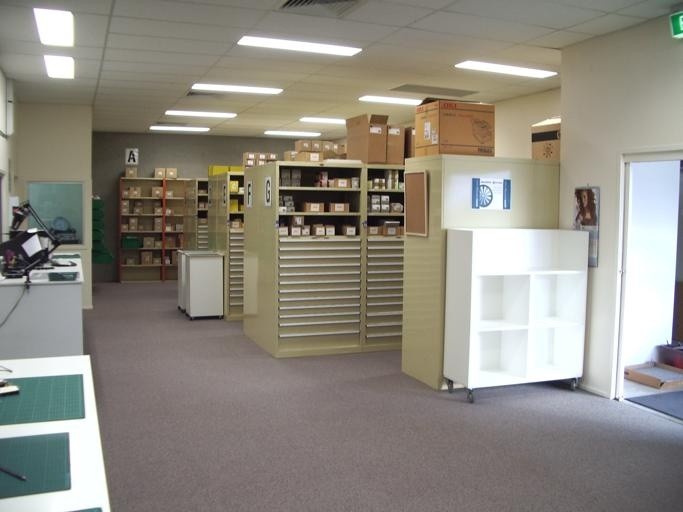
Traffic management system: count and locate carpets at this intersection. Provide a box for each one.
[625,391,682,421]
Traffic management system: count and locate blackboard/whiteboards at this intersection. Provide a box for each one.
[27,182,83,245]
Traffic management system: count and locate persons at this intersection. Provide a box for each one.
[575,189,597,225]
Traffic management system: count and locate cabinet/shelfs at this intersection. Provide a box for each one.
[175,172,243,325]
[399,157,561,396]
[116,179,188,282]
[242,162,404,360]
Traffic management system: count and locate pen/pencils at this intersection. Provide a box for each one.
[0,469,28,482]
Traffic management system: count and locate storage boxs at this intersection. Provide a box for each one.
[344,112,414,165]
[124,166,178,179]
[283,140,344,162]
[529,115,562,162]
[241,150,278,167]
[413,97,498,160]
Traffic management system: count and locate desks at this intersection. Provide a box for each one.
[0,251,112,512]
